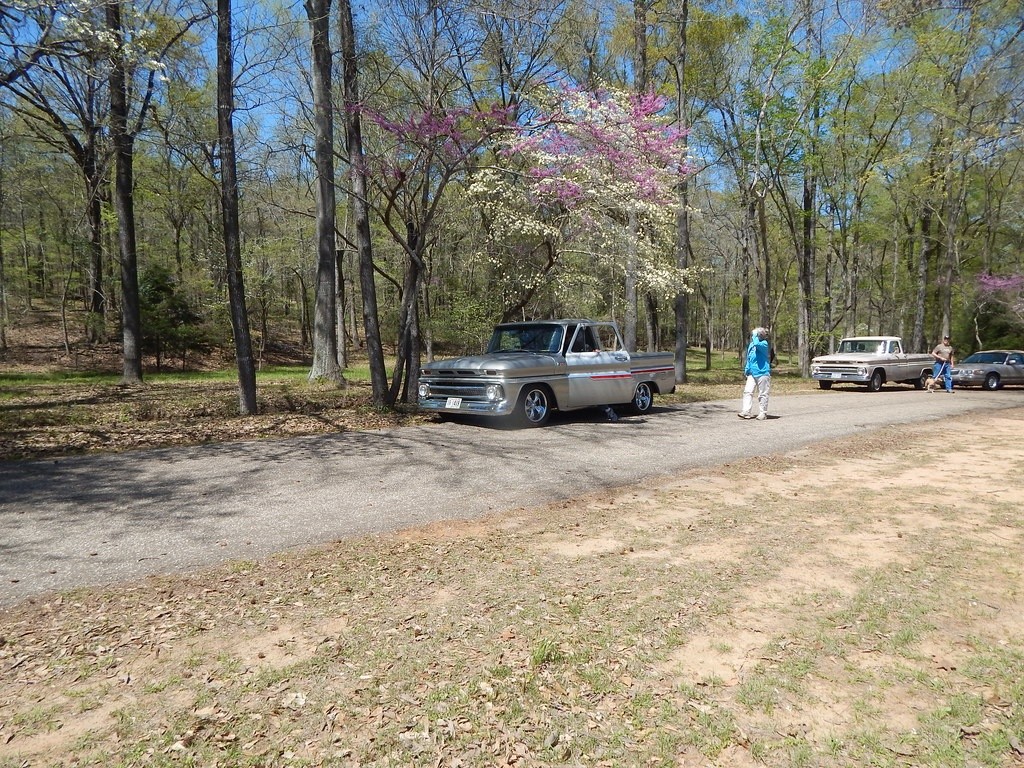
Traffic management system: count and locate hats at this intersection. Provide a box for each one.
[944,335,951,339]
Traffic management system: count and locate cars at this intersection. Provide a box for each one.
[950,350,1024,391]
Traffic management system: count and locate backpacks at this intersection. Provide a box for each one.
[770,347,779,369]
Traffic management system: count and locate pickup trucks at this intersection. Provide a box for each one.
[810,336,935,392]
[417,319,677,427]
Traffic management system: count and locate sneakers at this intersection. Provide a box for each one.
[756,414,767,420]
[947,390,955,393]
[738,412,750,419]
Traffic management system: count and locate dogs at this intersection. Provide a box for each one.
[923,378,942,392]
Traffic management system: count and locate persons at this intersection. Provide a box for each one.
[565,331,618,422]
[933,336,955,393]
[737,327,770,420]
